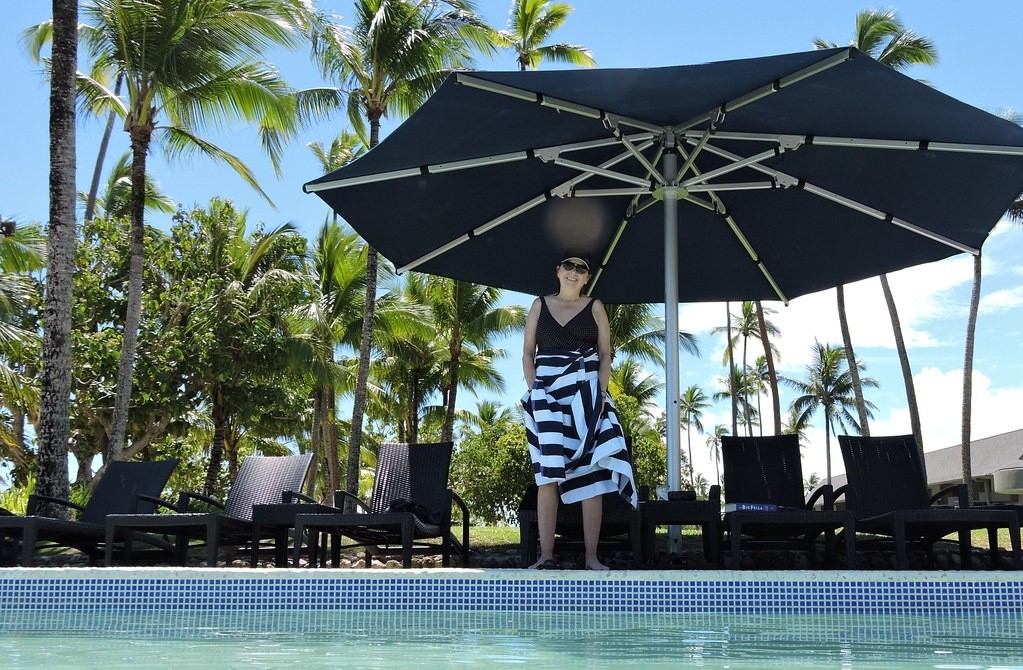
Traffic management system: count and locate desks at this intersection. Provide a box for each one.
[248,503,341,567]
[646,499,720,570]
[984,505,1023,570]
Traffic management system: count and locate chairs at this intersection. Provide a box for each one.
[708,433,856,573]
[830,434,1023,573]
[291,441,469,569]
[0,455,181,566]
[517,438,649,569]
[106,452,313,567]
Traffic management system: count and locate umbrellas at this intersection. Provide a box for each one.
[302,45,1023,552]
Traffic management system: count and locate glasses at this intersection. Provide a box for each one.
[559,260,592,275]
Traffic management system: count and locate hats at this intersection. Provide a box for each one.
[561,246,592,270]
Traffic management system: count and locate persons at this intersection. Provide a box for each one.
[520,246,638,569]
